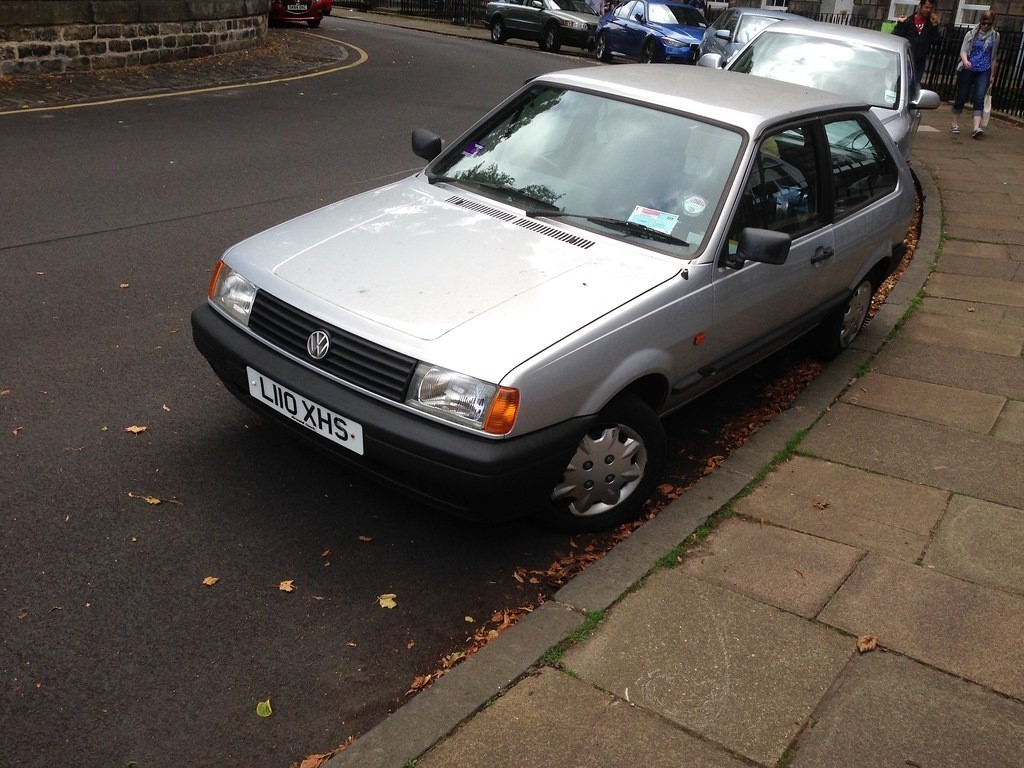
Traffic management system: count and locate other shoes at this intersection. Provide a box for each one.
[972,128,983,137]
[950,123,960,133]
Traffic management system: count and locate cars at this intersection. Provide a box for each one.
[595,0,711,65]
[483,0,604,53]
[695,18,941,163]
[694,5,815,72]
[267,1,335,29]
[187,60,920,540]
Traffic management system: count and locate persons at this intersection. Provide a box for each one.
[894,0,944,83]
[760,137,780,160]
[948,10,1000,138]
[581,0,605,52]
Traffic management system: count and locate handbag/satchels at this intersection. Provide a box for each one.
[981,83,991,127]
[880,18,898,34]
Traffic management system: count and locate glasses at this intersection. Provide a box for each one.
[981,22,993,26]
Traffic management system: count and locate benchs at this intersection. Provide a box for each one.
[774,135,879,204]
